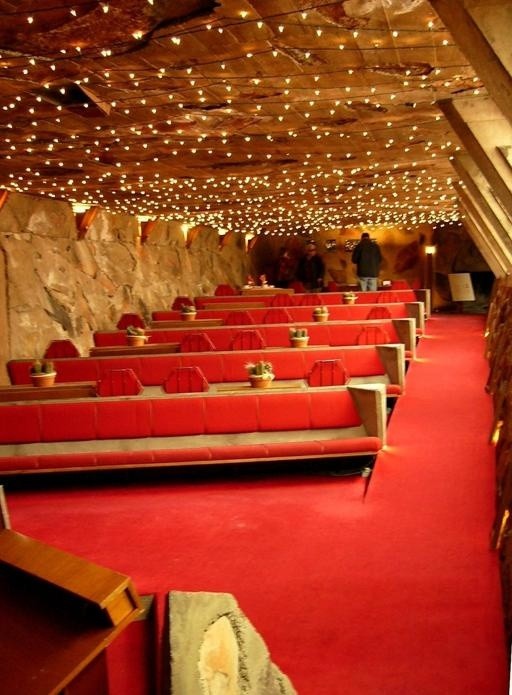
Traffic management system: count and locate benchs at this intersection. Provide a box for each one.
[1,283,432,478]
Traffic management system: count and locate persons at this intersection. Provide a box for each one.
[352,233,380,291]
[273,246,294,288]
[294,243,325,293]
